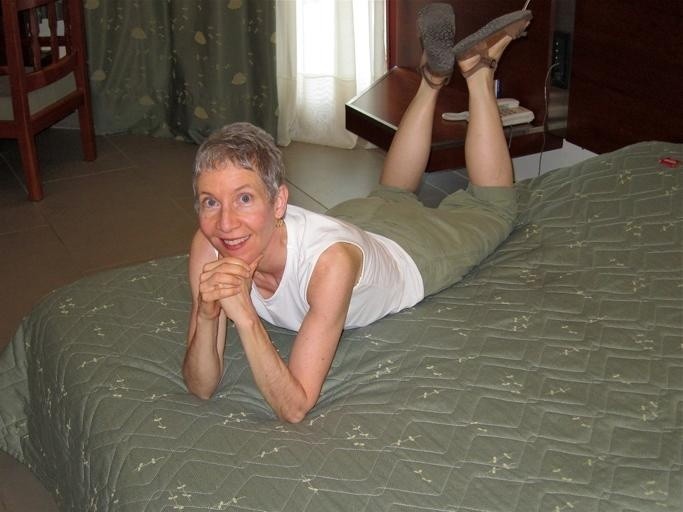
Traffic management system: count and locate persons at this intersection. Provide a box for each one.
[179,1,535,426]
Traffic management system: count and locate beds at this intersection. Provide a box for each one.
[0,139,683,512]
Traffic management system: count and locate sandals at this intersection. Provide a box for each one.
[416,2,459,92]
[450,7,534,81]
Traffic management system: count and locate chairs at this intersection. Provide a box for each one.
[0,0,98,202]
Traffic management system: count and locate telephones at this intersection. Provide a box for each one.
[466,99,534,127]
